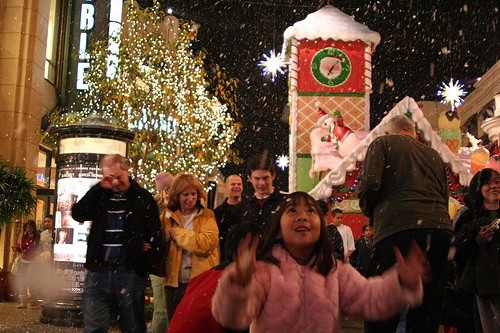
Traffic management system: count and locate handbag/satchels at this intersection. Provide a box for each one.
[11,249,23,274]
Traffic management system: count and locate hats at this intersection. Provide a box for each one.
[155,172,174,191]
[23,220,36,231]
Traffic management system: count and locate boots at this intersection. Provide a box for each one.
[28,289,38,307]
[16,295,28,308]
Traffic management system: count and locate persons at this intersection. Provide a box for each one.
[214,175,247,261]
[329,208,355,263]
[143,174,220,321]
[318,199,345,263]
[71,154,161,333]
[358,115,454,333]
[246,159,284,261]
[349,223,374,278]
[432,168,500,333]
[11,215,52,309]
[212,191,423,333]
[150,171,173,333]
